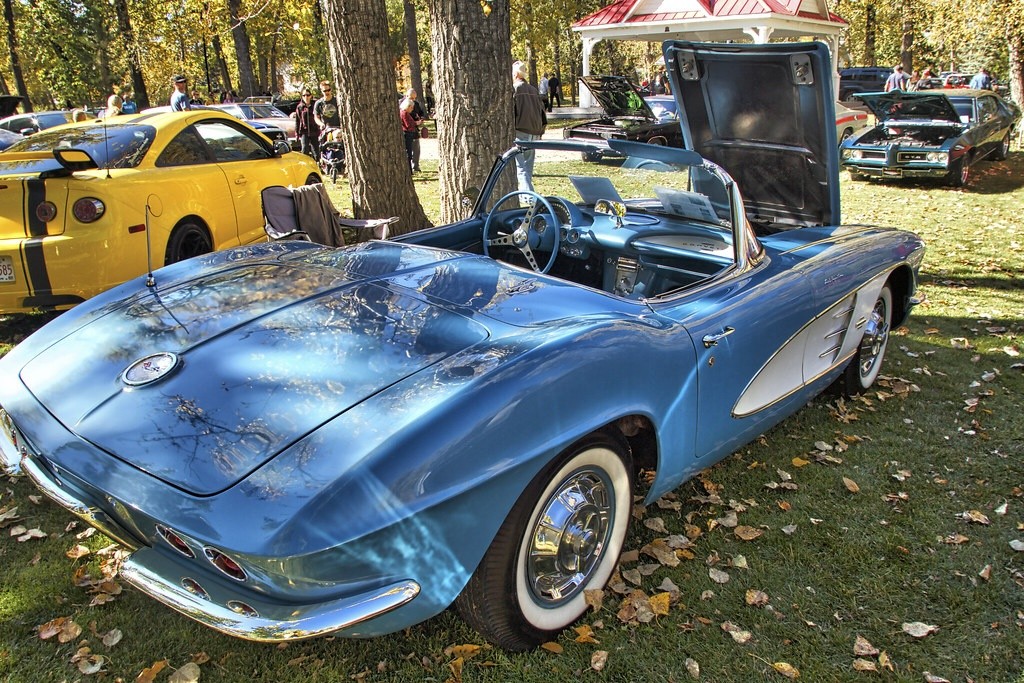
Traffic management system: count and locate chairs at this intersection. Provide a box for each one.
[260,184,399,246]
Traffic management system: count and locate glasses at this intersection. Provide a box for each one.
[302,95,310,96]
[176,80,186,83]
[323,88,331,92]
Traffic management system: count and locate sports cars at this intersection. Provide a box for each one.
[0,109,324,315]
[140,105,293,151]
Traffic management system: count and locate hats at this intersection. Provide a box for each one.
[512,61,525,78]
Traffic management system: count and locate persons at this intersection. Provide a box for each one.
[969,67,999,94]
[312,81,347,174]
[256,85,272,96]
[294,88,322,172]
[98,91,139,118]
[190,89,245,105]
[170,75,192,111]
[512,61,546,206]
[539,72,561,112]
[425,82,433,114]
[399,88,425,175]
[642,66,671,95]
[75,112,86,122]
[884,65,937,92]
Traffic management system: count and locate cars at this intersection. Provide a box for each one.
[945,74,1009,97]
[0,111,100,151]
[207,103,302,147]
[562,74,686,163]
[834,101,868,147]
[838,87,1023,186]
[0,38,926,657]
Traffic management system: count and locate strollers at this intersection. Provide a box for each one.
[318,127,345,184]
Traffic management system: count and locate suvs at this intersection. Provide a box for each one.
[838,67,944,104]
